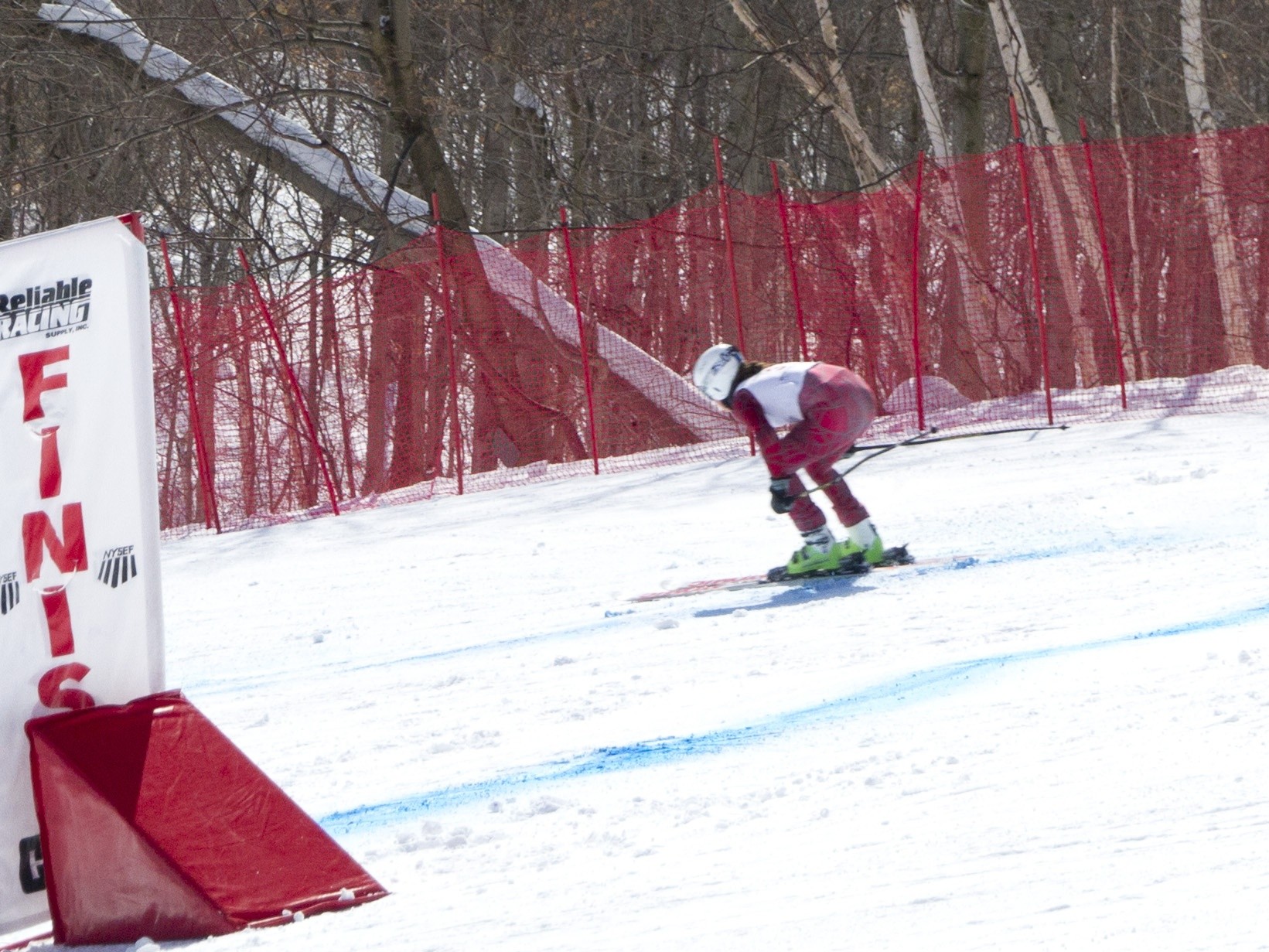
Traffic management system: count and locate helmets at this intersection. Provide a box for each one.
[692,343,740,401]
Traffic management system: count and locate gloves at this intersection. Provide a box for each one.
[768,477,796,513]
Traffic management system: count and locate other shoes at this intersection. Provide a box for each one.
[837,538,884,565]
[786,546,841,574]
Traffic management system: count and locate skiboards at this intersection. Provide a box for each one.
[623,547,1009,604]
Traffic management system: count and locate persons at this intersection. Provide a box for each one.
[692,343,883,575]
